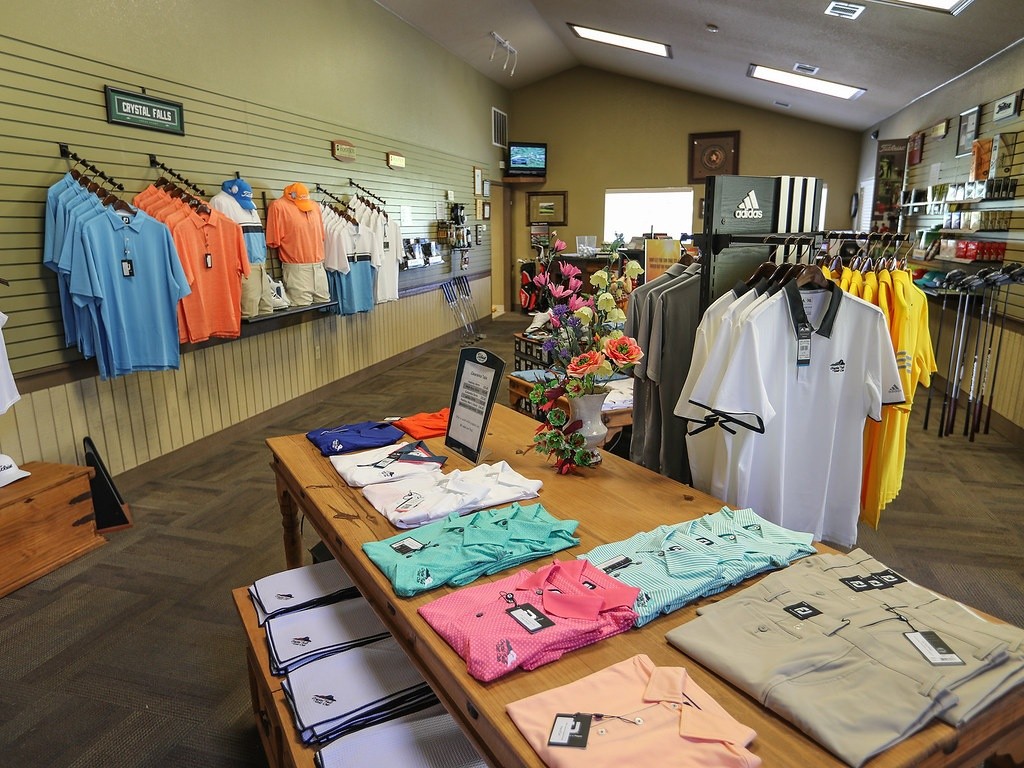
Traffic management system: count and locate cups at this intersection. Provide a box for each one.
[576,236,586,256]
[584,236,597,258]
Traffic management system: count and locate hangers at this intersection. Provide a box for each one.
[353,183,391,220]
[674,232,706,272]
[743,233,915,294]
[153,159,215,218]
[317,188,363,230]
[62,147,139,219]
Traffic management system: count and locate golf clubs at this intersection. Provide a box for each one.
[440,274,488,348]
[921,259,1023,441]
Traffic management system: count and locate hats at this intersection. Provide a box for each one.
[283,182,314,211]
[912,269,930,280]
[221,178,257,210]
[914,271,946,286]
[0,452,32,489]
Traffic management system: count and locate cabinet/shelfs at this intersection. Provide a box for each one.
[228,364,1023,767]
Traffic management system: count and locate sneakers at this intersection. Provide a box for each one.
[525,307,554,333]
[267,272,290,310]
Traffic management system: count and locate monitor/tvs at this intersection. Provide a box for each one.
[508,142,547,171]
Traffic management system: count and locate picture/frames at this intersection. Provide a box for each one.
[685,128,741,184]
[524,188,570,228]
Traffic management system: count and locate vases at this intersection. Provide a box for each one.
[563,383,610,463]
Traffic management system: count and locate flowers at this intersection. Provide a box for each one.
[515,228,646,476]
